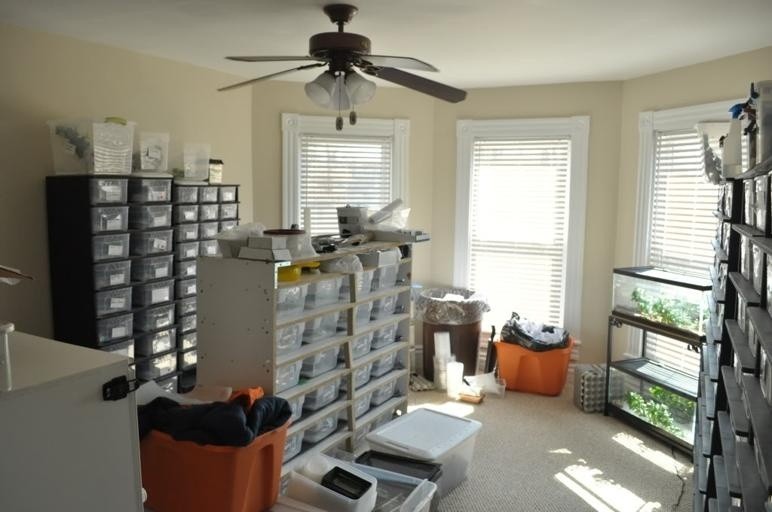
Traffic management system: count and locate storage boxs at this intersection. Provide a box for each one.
[493,337,575,396]
[209,159,224,182]
[171,138,210,181]
[694,80,771,511]
[365,407,483,498]
[132,128,170,172]
[45,116,137,174]
[573,363,624,413]
[140,416,292,512]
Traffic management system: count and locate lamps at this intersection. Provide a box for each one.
[304,67,377,131]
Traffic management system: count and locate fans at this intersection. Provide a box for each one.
[216,4,468,104]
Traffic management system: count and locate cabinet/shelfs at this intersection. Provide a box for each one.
[195,240,414,498]
[0,331,145,512]
[692,153,772,511]
[604,267,713,464]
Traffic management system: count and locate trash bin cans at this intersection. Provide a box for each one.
[420,286,481,382]
